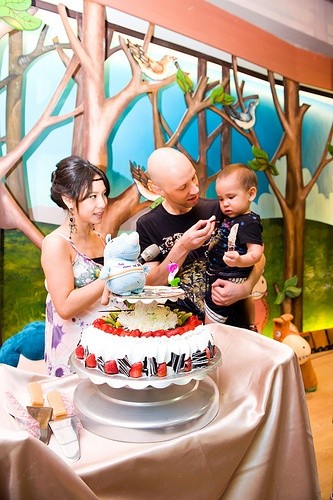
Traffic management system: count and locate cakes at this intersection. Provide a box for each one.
[76,299,216,378]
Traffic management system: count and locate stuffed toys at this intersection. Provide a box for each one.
[99,232,150,296]
[273,313,318,392]
[0,322,45,368]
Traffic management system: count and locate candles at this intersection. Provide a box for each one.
[168,261,180,288]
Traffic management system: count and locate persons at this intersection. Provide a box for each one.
[136,148,266,330]
[41,155,110,379]
[204,163,265,325]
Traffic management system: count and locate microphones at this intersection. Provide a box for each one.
[138,244,160,265]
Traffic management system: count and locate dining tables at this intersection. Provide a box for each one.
[0,324,322,500]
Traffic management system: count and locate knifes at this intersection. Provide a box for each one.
[227,223,239,252]
